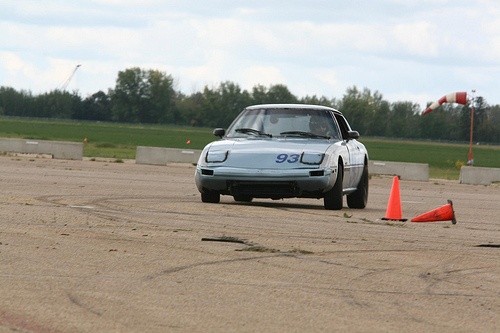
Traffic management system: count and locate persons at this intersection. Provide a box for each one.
[309,116,337,139]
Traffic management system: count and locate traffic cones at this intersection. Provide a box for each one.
[382,175,407,221]
[411,200,457,226]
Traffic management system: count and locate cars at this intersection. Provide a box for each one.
[194,103,370,212]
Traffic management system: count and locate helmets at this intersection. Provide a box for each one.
[309,115,329,135]
